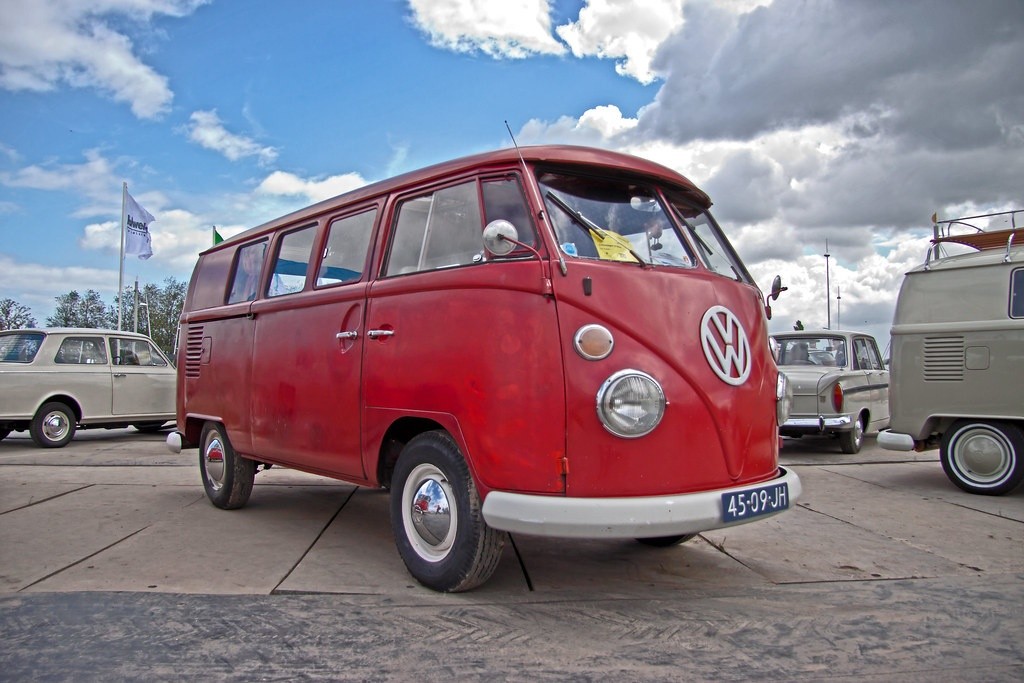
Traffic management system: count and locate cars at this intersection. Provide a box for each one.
[770,330,890,454]
[0,328,177,448]
[85,348,140,365]
[2,346,36,361]
[152,353,176,364]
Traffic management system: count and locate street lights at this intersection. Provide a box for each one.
[836,286,842,330]
[822,238,830,330]
[131,303,147,357]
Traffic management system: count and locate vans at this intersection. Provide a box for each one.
[167,145,802,593]
[876,209,1024,495]
